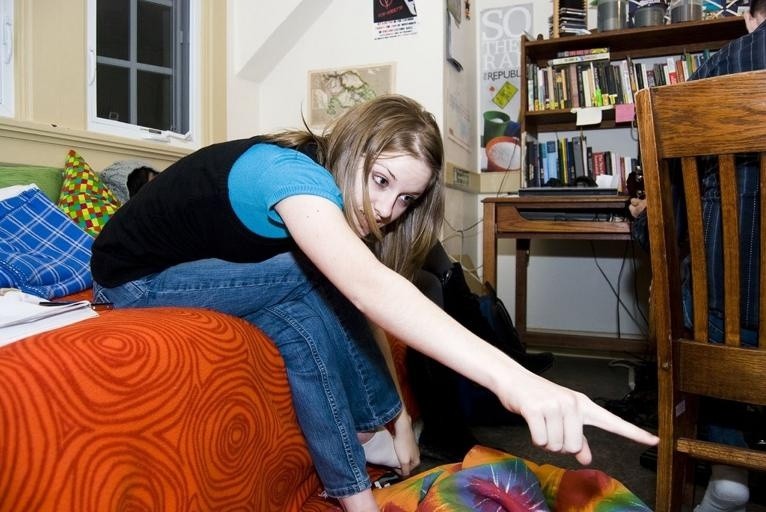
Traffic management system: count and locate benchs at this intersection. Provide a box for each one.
[0,288,417,510]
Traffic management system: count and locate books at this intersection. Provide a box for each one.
[520,44,713,197]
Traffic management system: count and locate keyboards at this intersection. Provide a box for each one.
[518,210,610,222]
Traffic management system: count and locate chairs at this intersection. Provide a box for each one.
[634,69,766,509]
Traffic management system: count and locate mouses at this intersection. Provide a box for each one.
[613,212,625,223]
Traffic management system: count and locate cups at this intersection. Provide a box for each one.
[483,110,521,171]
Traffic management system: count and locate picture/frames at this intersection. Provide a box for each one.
[307,62,395,129]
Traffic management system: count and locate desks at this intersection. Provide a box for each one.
[480,15,752,361]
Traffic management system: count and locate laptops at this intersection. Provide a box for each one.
[518,185,620,195]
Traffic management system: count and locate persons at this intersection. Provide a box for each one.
[622,0,765,512]
[87,94,663,511]
